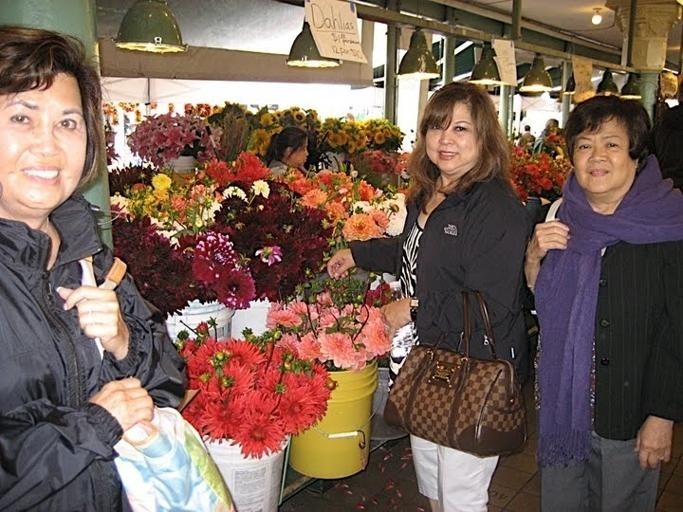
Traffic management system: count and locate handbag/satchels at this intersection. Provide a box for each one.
[383,344,526,458]
[112,406,236,512]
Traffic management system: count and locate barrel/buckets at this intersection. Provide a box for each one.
[289,356,383,479]
[164,300,236,347]
[370,368,413,440]
[198,433,289,511]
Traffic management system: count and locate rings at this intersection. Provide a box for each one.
[658,454,664,460]
[86,309,95,326]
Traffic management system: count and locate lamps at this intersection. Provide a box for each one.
[286,6,345,68]
[396,16,641,101]
[112,1,186,54]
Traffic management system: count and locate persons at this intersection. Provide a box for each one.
[537,114,561,157]
[262,125,312,175]
[0,25,187,511]
[517,125,536,148]
[525,96,682,512]
[649,78,682,192]
[325,80,532,512]
